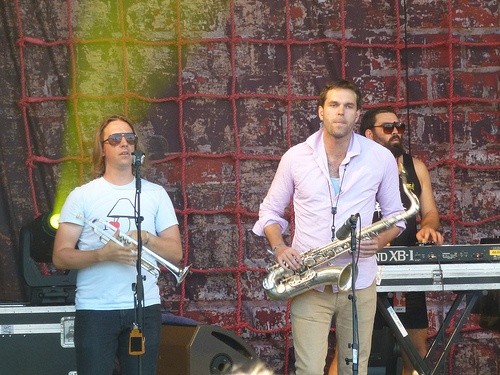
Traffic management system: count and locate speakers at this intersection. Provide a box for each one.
[157,324,269,375]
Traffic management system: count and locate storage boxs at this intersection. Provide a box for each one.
[0,303,77,375]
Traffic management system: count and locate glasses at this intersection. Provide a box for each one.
[372,120,406,135]
[101,130,138,144]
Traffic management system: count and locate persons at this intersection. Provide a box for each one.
[51,115,183,375]
[252,78,407,375]
[329,108,444,375]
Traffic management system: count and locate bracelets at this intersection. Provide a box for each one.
[272,242,284,252]
[144,231,149,246]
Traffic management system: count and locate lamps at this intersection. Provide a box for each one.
[19,210,78,305]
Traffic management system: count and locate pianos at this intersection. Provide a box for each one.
[376,244,500,264]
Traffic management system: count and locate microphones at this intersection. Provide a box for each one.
[131,150,145,167]
[336,213,359,240]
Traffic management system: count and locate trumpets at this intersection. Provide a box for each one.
[74,214,193,284]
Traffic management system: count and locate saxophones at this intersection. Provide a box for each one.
[262,170,421,300]
